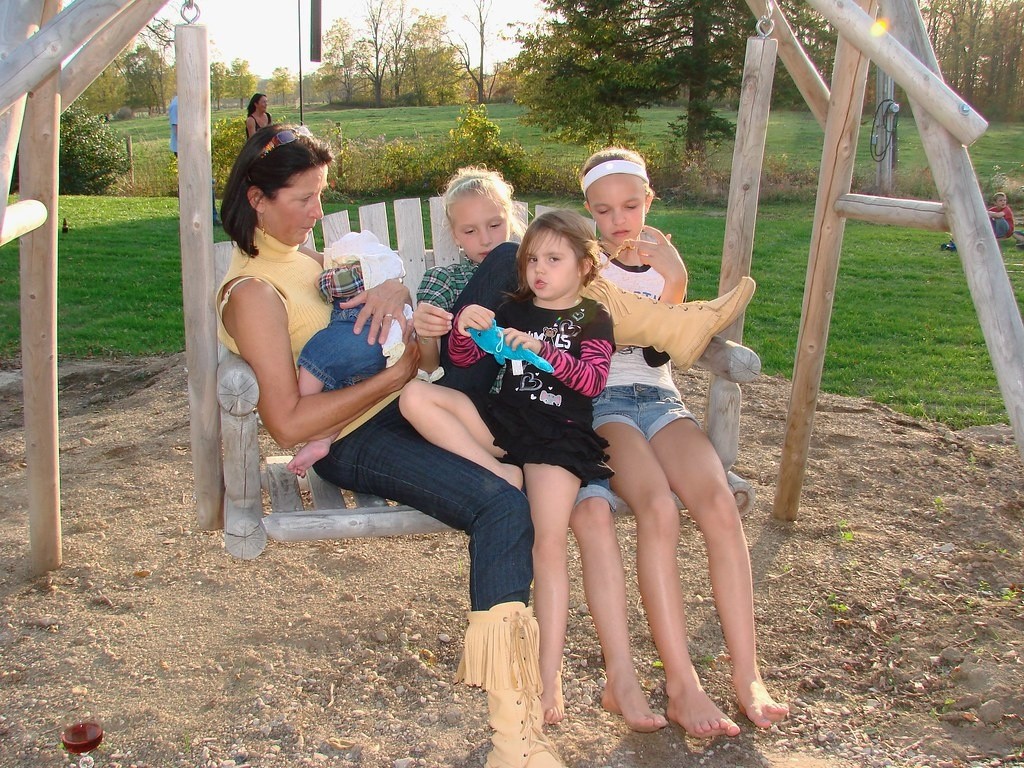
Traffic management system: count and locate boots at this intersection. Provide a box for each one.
[456,601,567,768]
[580,240,756,371]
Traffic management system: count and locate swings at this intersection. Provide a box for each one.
[167,6,784,559]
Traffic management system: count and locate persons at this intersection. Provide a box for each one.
[580,144,790,738]
[287,230,413,480]
[168,94,222,226]
[245,93,273,139]
[399,166,672,731]
[984,192,1024,247]
[217,123,757,768]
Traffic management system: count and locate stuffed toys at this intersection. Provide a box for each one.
[464,318,554,376]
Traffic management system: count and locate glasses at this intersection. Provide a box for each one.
[248,126,311,183]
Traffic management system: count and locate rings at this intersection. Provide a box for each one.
[384,314,393,317]
